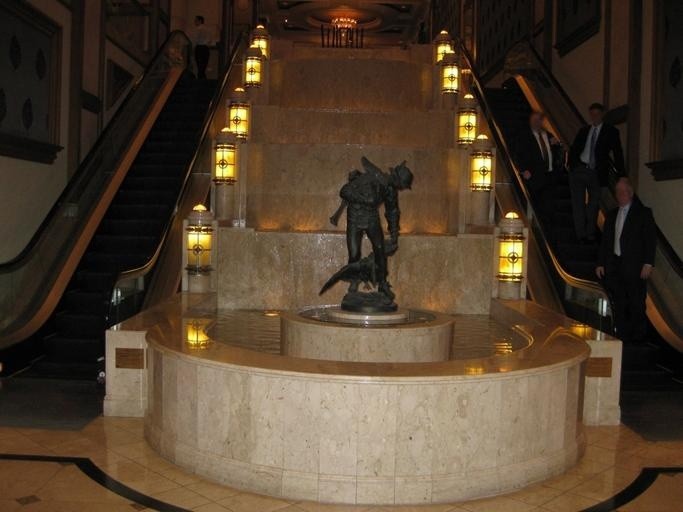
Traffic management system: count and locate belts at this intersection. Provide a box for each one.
[579,160,589,170]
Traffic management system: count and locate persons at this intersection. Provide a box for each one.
[183,15,217,81]
[593,176,658,346]
[514,111,559,227]
[566,103,628,244]
[338,156,414,299]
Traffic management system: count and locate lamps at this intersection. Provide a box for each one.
[435,27,524,282]
[330,17,358,36]
[185,24,269,275]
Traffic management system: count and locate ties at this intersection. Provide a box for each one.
[615,208,625,257]
[537,132,549,171]
[588,126,598,171]
